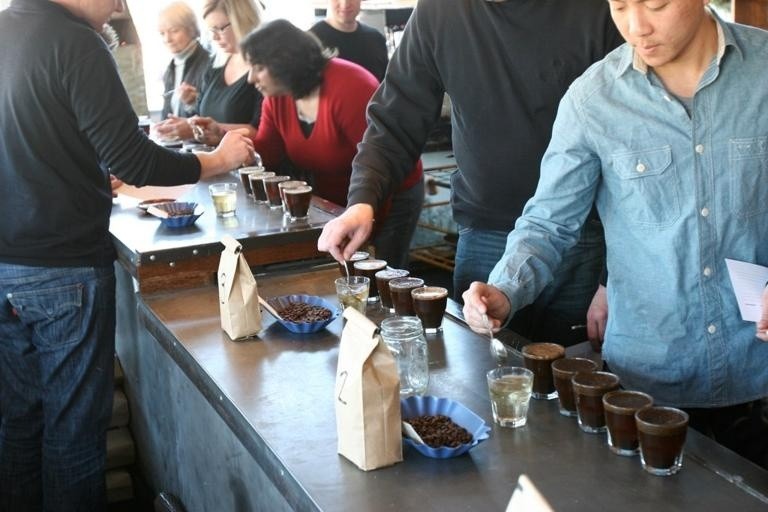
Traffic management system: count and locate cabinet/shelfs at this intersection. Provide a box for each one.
[408,164,459,271]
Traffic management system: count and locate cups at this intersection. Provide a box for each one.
[486,342,689,476]
[153,136,214,154]
[238,166,313,221]
[138,115,150,136]
[209,183,237,217]
[334,250,447,335]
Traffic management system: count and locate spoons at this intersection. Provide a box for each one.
[482,313,509,364]
[252,151,264,166]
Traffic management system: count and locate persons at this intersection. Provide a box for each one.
[184,19,424,267]
[157,0,263,141]
[307,0,389,82]
[316,0,627,353]
[462,0,767,467]
[156,3,208,119]
[0,0,259,510]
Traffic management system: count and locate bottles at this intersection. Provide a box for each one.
[381,317,428,395]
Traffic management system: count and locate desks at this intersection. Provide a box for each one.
[120,255,764,511]
[104,129,357,447]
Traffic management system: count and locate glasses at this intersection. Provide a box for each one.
[215,23,230,32]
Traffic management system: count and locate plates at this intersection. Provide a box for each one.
[135,198,176,214]
[264,295,339,335]
[149,202,205,229]
[399,397,490,461]
